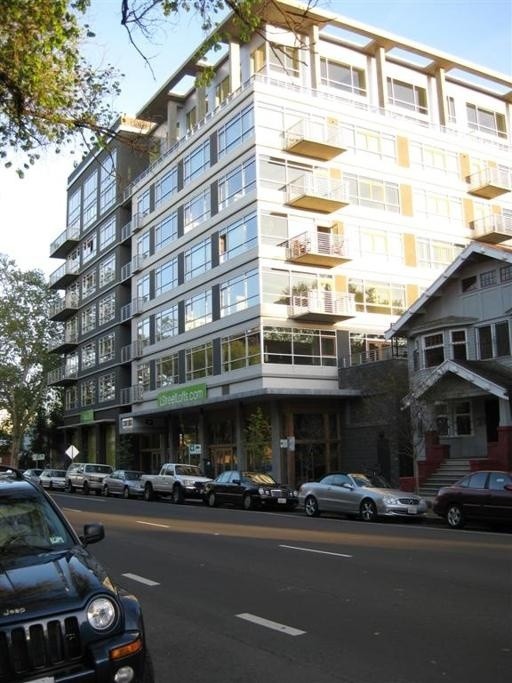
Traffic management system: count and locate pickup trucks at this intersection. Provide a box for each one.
[139,463,214,505]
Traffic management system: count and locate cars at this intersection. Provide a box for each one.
[38,468,68,491]
[23,468,44,481]
[101,469,149,499]
[297,469,429,523]
[201,470,299,513]
[432,468,512,533]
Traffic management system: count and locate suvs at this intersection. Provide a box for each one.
[0,464,156,682]
[64,462,114,496]
